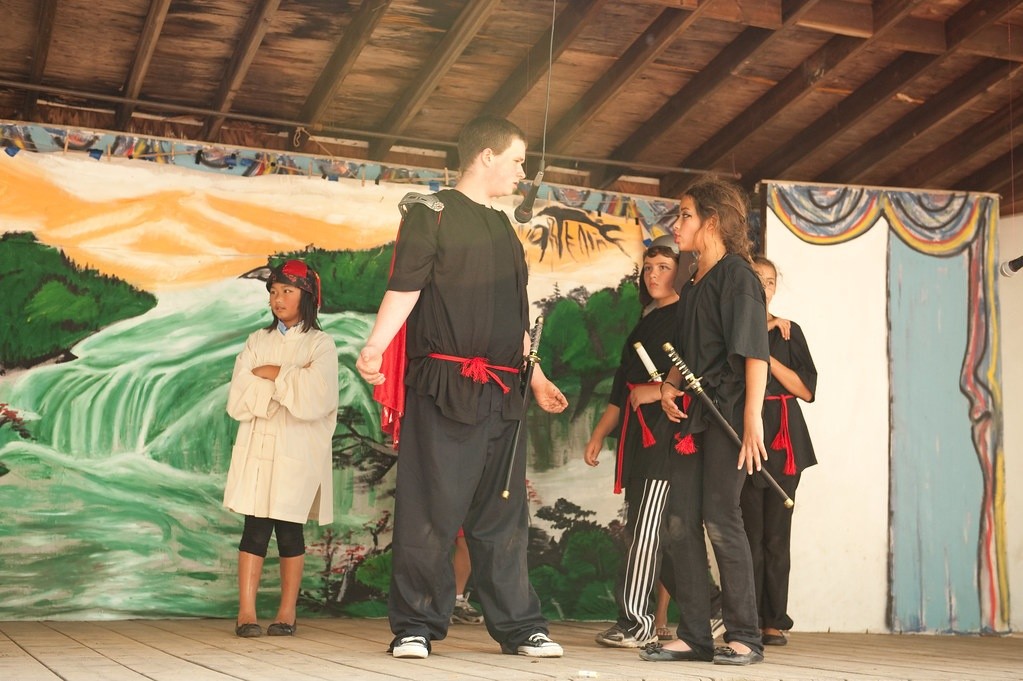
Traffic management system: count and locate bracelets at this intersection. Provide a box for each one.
[660,382,676,393]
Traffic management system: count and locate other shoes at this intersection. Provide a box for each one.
[640,641,701,660]
[762,629,787,644]
[712,645,763,665]
[234,621,261,637]
[268,620,297,635]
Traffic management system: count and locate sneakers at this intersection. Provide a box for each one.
[594,619,658,648]
[392,635,429,658]
[502,631,564,657]
[449,592,484,624]
[709,608,727,639]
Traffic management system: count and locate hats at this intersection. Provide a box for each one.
[266,260,320,308]
[639,234,697,305]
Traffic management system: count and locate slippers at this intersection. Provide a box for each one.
[656,626,673,640]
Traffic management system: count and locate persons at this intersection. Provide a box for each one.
[638,176,770,665]
[355,115,568,658]
[221,260,339,638]
[584,234,727,647]
[741,256,818,647]
